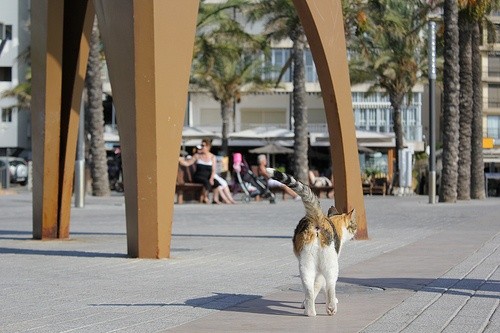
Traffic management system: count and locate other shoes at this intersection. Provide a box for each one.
[204,199,207,203]
[294,196,302,201]
[225,200,235,204]
[212,201,219,203]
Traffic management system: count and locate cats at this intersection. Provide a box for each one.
[260,164,360,318]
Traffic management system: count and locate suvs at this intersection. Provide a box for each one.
[1,157,28,187]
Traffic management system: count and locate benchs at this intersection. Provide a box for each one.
[175,162,204,203]
[311,187,333,198]
[372,182,386,196]
[362,183,373,196]
